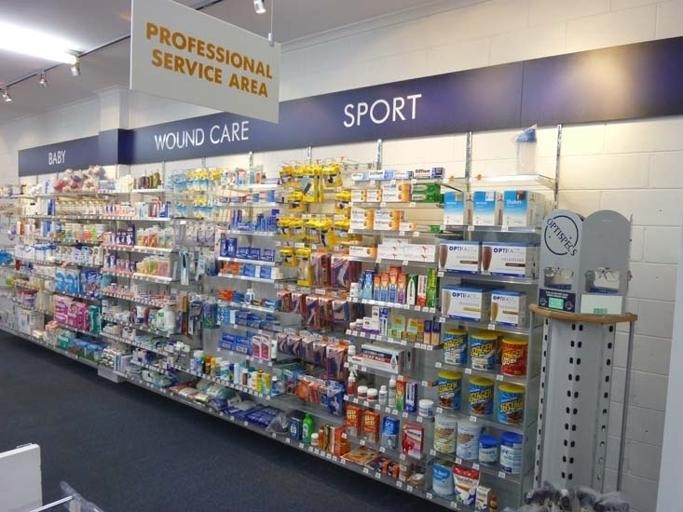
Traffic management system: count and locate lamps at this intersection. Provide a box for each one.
[0,21,84,66]
[0,0,269,103]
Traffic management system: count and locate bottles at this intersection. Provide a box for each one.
[378,383,386,406]
[387,376,396,409]
